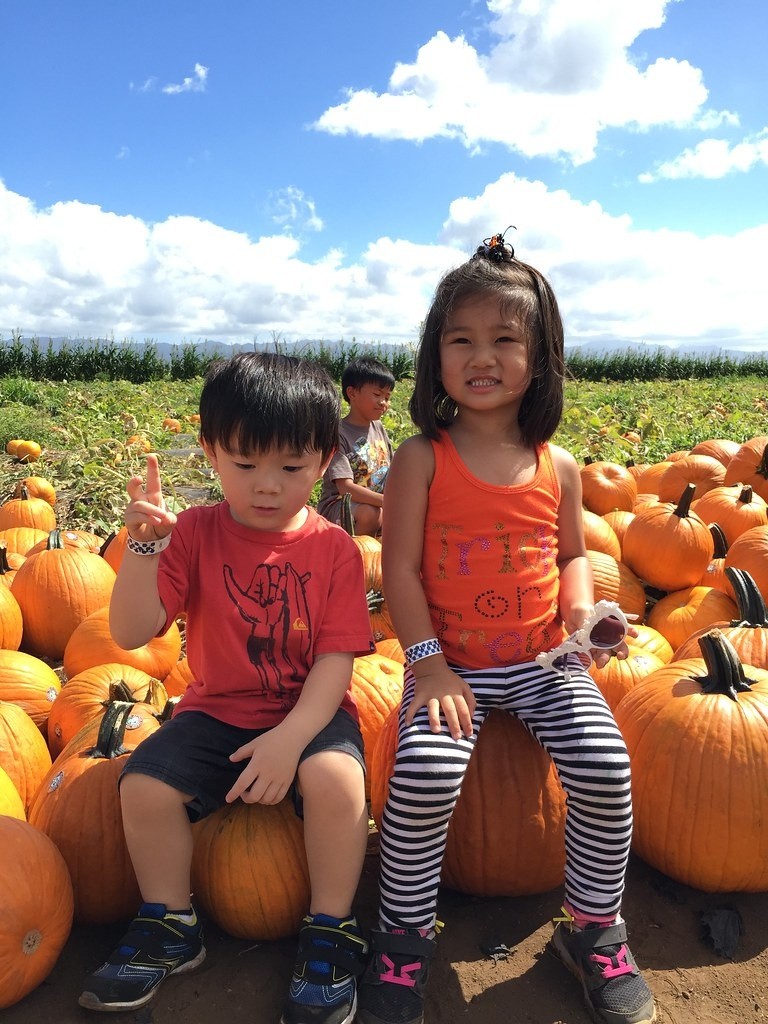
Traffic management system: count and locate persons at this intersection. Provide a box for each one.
[358,233,655,1024]
[78,352,378,1024]
[318,358,396,537]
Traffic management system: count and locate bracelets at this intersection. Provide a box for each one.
[127,530,172,556]
[403,638,443,668]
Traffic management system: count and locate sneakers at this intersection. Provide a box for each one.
[550,914,657,1024]
[357,921,445,1024]
[78,903,206,1010]
[281,913,369,1024]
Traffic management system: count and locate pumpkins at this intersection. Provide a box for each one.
[0,411,768,1013]
